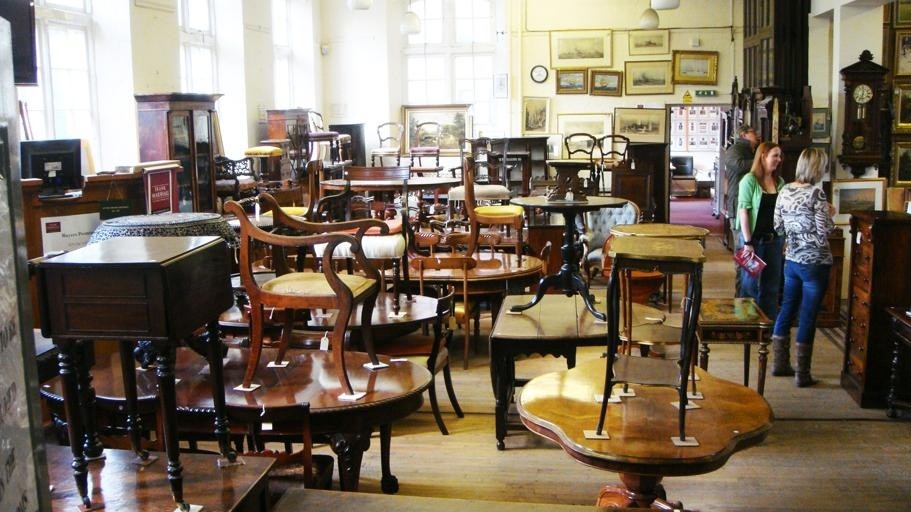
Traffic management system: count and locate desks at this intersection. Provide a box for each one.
[504,189,630,324]
[881,305,911,421]
[35,233,241,512]
[482,287,776,456]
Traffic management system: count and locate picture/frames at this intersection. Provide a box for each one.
[829,175,887,225]
[400,102,472,157]
[521,22,720,164]
[888,0,911,189]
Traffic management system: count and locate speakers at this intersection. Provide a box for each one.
[1,0,37,86]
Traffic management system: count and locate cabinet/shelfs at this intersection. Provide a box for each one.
[16,163,183,351]
[613,165,651,223]
[133,89,224,218]
[839,203,911,421]
[329,121,366,167]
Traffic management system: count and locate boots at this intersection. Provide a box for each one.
[772,335,795,376]
[795,342,820,386]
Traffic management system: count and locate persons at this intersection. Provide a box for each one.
[723,123,760,294]
[769,144,837,388]
[734,140,784,319]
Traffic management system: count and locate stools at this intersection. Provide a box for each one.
[242,124,350,189]
[594,219,710,439]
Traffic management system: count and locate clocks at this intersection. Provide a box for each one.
[838,49,892,166]
[529,65,549,84]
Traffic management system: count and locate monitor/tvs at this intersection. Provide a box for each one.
[20,138,81,200]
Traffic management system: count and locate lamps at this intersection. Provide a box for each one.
[637,0,660,29]
